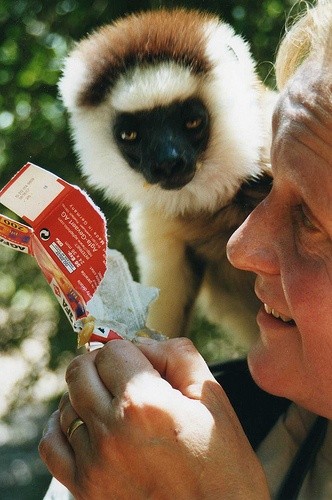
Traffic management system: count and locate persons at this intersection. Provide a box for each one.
[40,1,332,499]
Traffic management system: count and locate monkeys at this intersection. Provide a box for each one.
[56,9,281,355]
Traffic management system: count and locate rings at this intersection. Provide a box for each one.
[65,416,86,442]
